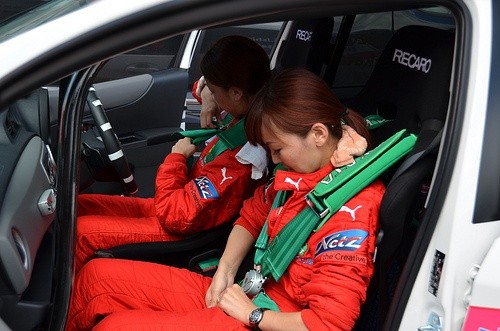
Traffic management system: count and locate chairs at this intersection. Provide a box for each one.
[96,16,334,265]
[200,25,455,331]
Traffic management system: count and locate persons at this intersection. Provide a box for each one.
[66,67,387,331]
[73,35,275,277]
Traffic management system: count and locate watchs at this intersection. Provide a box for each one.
[248,307,271,331]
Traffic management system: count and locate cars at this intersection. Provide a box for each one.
[1,0,500,330]
